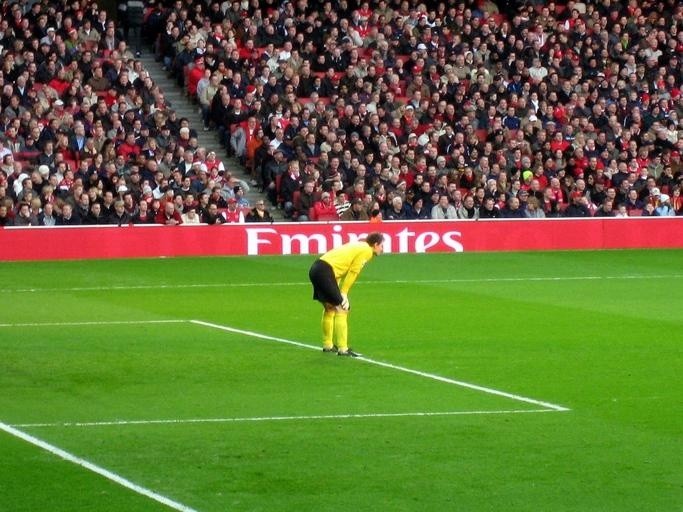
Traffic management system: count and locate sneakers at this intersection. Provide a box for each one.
[323,344,338,352]
[339,348,361,356]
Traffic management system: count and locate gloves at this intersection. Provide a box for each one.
[340,293,349,310]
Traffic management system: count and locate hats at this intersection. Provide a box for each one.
[518,190,529,196]
[46,26,76,34]
[444,64,452,72]
[418,31,439,50]
[651,187,670,203]
[227,198,237,203]
[449,41,473,58]
[393,179,441,206]
[404,105,417,138]
[143,185,151,194]
[437,156,445,164]
[55,99,63,105]
[39,165,49,175]
[529,115,555,125]
[597,72,618,77]
[520,157,533,180]
[118,185,127,191]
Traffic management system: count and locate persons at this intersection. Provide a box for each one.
[2,2,683,229]
[307,231,385,359]
